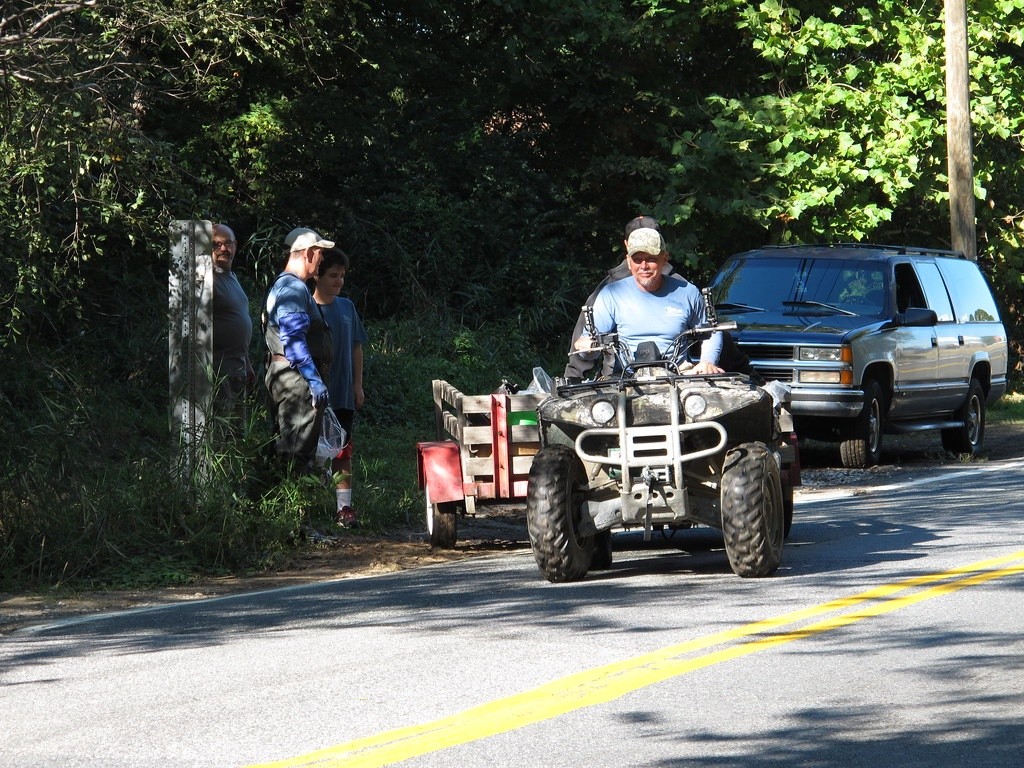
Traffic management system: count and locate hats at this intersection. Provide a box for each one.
[626,216,659,234]
[284,227,335,255]
[627,228,666,255]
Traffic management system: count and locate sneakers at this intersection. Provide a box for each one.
[335,506,358,527]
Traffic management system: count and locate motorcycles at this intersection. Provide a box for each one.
[525,319,786,578]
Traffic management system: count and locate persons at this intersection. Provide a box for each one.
[212,223,364,530]
[564,216,766,387]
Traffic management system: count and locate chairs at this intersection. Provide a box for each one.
[863,288,908,314]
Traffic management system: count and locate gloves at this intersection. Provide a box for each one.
[277,312,330,413]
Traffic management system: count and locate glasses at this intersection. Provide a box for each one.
[212,240,235,251]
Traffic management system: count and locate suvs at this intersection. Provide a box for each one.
[703,242,1010,467]
